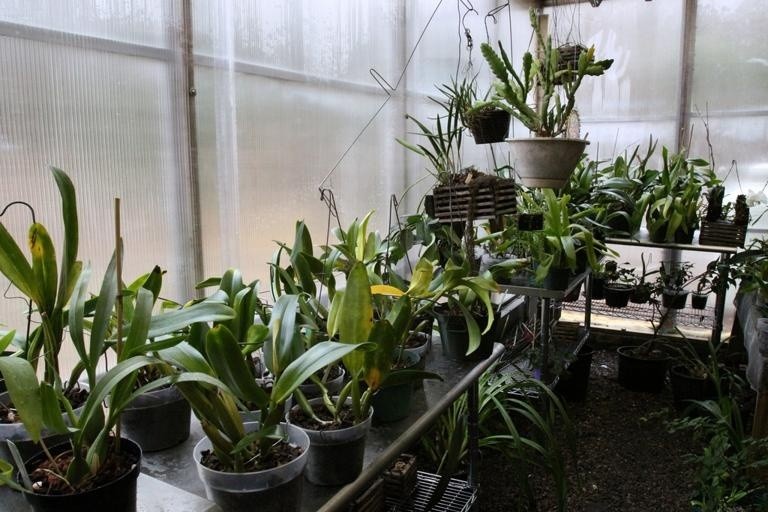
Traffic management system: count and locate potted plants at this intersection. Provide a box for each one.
[2,7,768,512]
